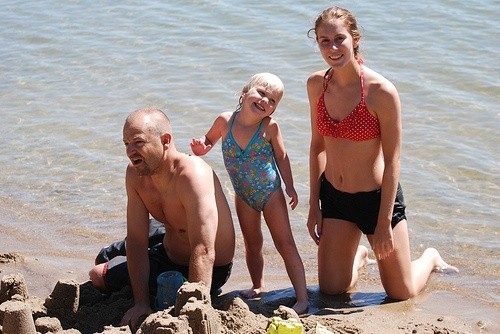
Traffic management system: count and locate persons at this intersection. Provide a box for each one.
[89,107,236,334]
[306,6,460,301]
[190,73,311,315]
